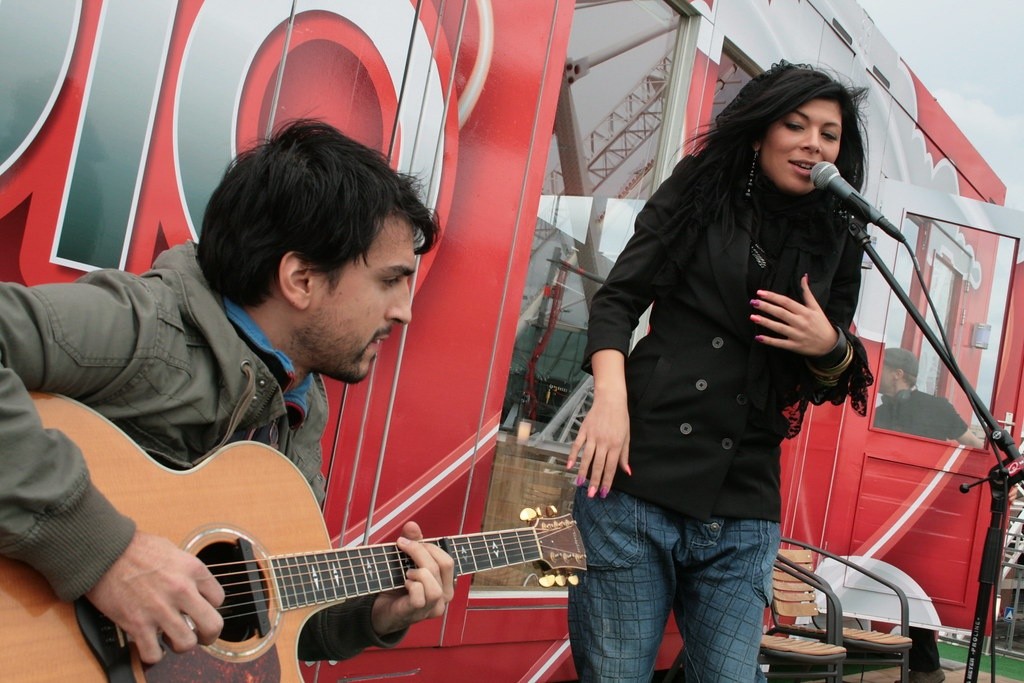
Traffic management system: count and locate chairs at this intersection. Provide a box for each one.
[758,561,847,683]
[776,538,912,683]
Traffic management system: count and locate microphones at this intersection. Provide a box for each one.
[810,162,906,243]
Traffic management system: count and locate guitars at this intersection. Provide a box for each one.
[0,389,589,683]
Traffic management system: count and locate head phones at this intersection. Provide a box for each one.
[881,384,918,411]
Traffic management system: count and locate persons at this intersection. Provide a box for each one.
[1,115,457,665]
[568,58,873,683]
[874,347,986,450]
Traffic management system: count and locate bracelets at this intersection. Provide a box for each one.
[806,340,854,388]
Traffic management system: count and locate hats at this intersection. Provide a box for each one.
[882,347,917,376]
